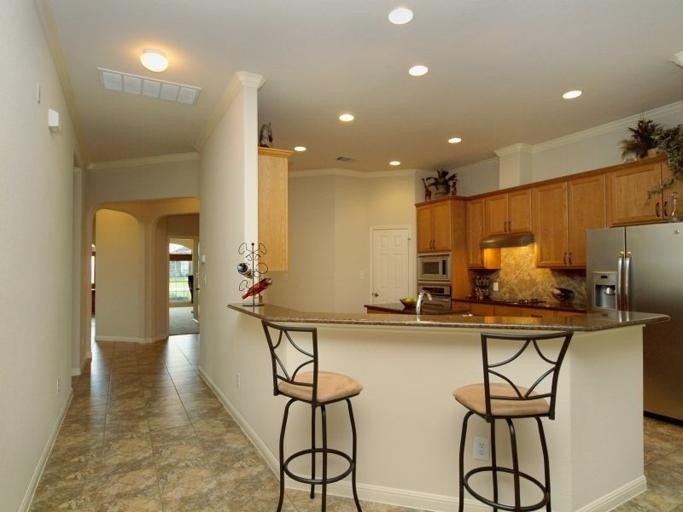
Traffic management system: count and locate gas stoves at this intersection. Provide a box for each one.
[497,294,544,311]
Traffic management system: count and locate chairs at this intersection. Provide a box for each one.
[453,330,575,512]
[261,317,367,512]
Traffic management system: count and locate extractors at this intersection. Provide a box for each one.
[475,227,538,249]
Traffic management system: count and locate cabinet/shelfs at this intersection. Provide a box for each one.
[485,183,534,267]
[258,147,294,273]
[415,201,466,251]
[608,155,682,221]
[536,166,606,269]
[466,196,484,269]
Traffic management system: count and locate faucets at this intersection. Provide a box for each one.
[416,290,432,315]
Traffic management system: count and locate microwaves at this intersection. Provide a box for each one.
[421,296,452,312]
[416,253,451,280]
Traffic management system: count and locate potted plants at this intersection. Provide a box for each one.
[426,168,457,197]
[621,122,662,160]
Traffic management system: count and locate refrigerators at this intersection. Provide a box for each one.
[586,220,683,421]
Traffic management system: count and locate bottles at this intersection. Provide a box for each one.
[237,263,253,280]
[241,278,272,299]
